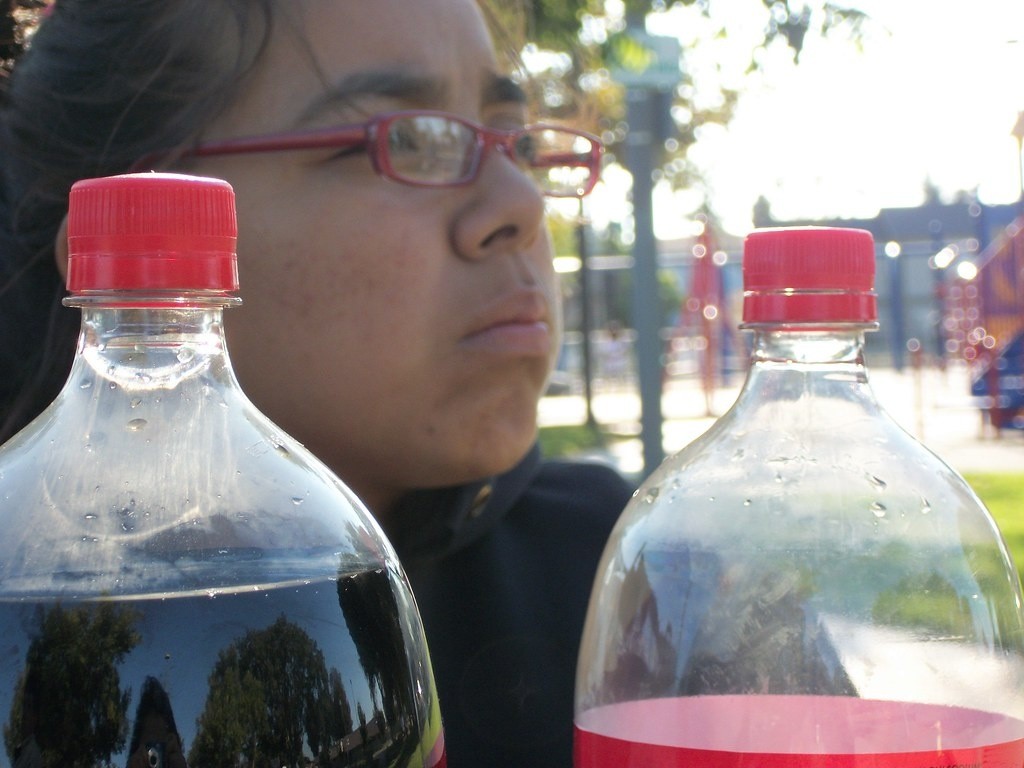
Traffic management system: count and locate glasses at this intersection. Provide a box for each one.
[121,110,605,197]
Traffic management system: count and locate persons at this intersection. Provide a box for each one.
[0,0,855,765]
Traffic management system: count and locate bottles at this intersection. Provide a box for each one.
[571,229,1024,767]
[2,172,446,766]
